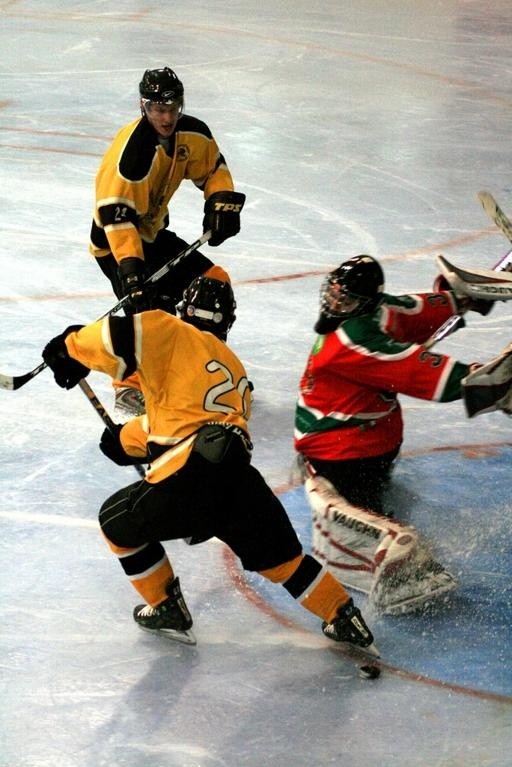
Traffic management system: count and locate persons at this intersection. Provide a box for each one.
[41,275,375,648]
[88,67,248,412]
[294,253,493,614]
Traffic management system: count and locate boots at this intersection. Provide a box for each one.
[321,598,374,648]
[134,577,192,630]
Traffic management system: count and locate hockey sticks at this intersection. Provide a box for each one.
[1,229,212,391]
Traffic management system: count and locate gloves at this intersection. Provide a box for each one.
[203,192,245,246]
[99,424,150,466]
[118,257,151,304]
[43,324,90,389]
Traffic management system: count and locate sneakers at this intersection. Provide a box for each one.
[385,565,456,617]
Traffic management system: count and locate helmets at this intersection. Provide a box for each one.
[314,255,384,334]
[177,276,235,341]
[140,66,184,119]
